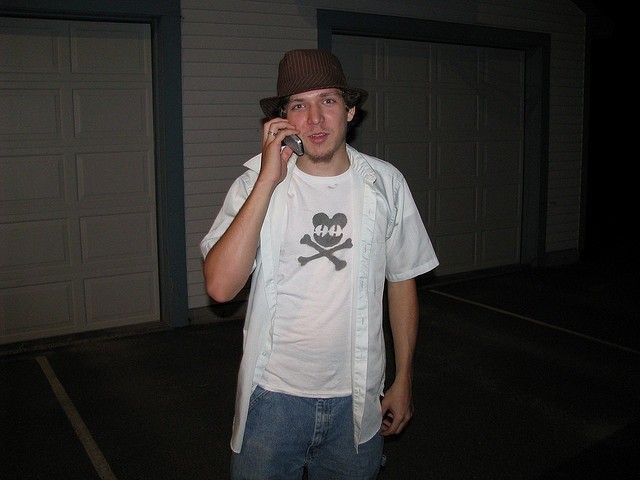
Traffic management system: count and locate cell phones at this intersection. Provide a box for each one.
[281,132,305,158]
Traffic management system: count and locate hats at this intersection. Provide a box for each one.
[259,50,371,121]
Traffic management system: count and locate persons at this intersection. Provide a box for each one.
[201,47,438,480]
